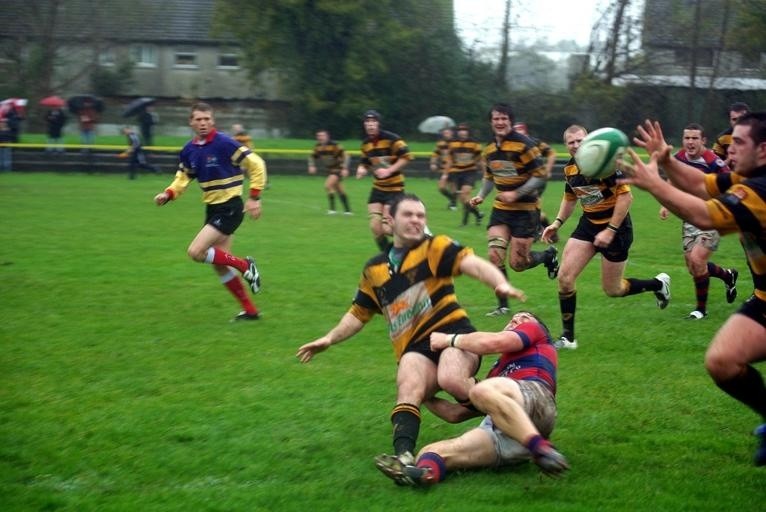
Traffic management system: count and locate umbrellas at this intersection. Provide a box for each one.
[1,95,29,116]
[38,95,65,108]
[67,92,107,115]
[122,94,157,118]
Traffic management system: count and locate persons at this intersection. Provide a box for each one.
[81,99,97,157]
[139,104,157,144]
[615,101,766,466]
[1,119,14,172]
[541,127,672,349]
[308,129,351,214]
[357,111,431,249]
[469,102,560,315]
[394,309,566,484]
[299,194,527,489]
[45,103,65,154]
[120,125,160,180]
[153,102,268,320]
[228,122,253,150]
[431,124,485,226]
[7,105,22,143]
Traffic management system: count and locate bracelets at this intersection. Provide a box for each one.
[450,331,460,346]
[249,196,262,202]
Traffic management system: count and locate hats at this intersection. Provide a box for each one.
[361,110,381,123]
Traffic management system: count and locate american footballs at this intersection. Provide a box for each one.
[570,127,631,179]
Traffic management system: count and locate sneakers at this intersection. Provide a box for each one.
[544,246,560,279]
[327,210,337,214]
[476,211,484,225]
[344,211,354,216]
[553,336,578,349]
[374,452,413,486]
[539,445,569,472]
[654,273,671,309]
[725,268,738,303]
[400,463,437,493]
[230,310,260,322]
[243,257,261,293]
[449,206,458,211]
[485,307,511,317]
[687,311,707,320]
